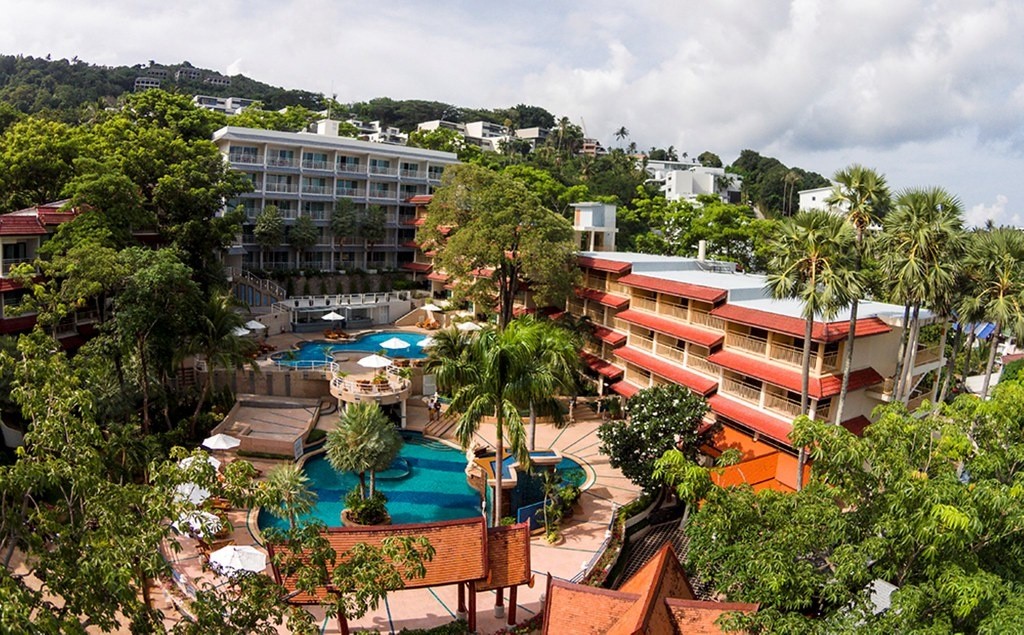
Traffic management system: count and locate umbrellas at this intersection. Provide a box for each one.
[380,338,411,349]
[321,311,345,322]
[357,354,391,368]
[417,338,438,346]
[209,545,266,578]
[177,454,220,475]
[420,304,442,311]
[174,510,222,538]
[245,320,266,330]
[173,481,210,505]
[232,327,250,336]
[457,322,483,330]
[202,433,241,464]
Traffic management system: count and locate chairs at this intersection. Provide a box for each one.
[323,327,349,339]
[243,336,274,359]
[195,458,263,562]
[416,318,441,329]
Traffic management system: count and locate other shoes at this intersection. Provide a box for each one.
[430,418,434,421]
[437,418,439,420]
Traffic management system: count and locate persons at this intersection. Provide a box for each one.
[428,399,435,421]
[434,400,441,420]
[264,325,270,335]
